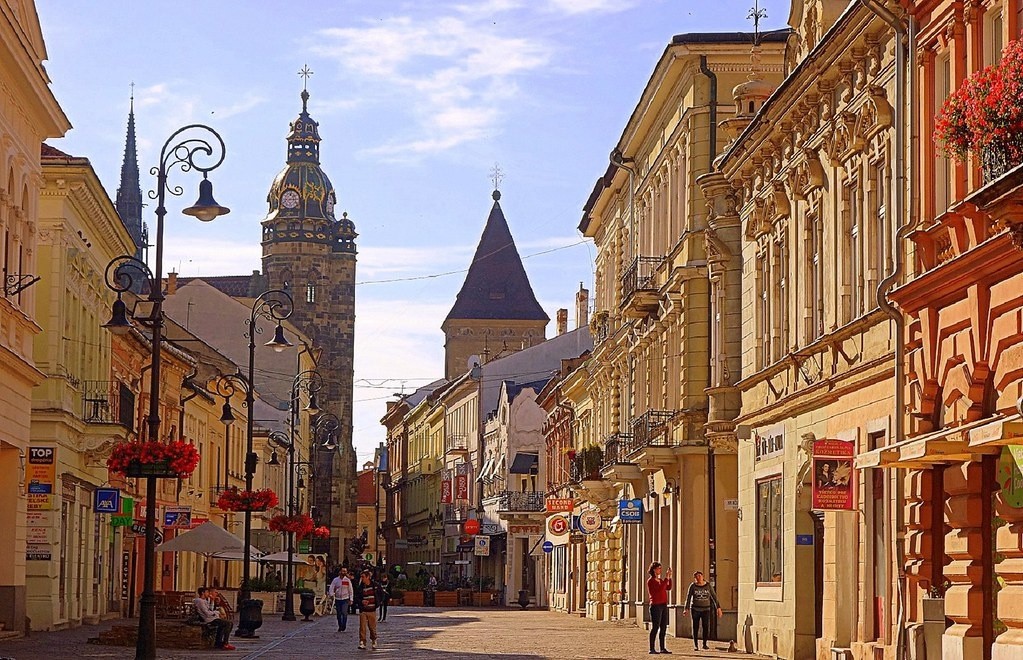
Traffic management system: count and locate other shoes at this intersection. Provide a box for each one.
[703,645,709,649]
[214,646,227,650]
[372,642,377,648]
[660,649,672,654]
[694,646,698,650]
[358,644,366,649]
[224,643,235,649]
[649,650,660,654]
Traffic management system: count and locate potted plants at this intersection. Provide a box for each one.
[389,574,499,606]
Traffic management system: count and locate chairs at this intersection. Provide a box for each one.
[153,590,196,618]
[308,592,339,617]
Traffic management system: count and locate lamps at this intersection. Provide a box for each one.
[663,477,679,499]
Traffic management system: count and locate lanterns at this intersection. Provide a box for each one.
[464,520,480,535]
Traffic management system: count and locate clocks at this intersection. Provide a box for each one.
[326,194,334,212]
[281,191,300,209]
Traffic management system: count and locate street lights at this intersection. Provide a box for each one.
[267,369,324,624]
[100,124,231,660]
[288,412,343,552]
[217,288,295,606]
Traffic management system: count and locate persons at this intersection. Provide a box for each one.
[376,574,391,623]
[818,462,833,488]
[329,566,353,631]
[683,571,723,651]
[646,562,672,654]
[431,572,437,587]
[302,555,326,581]
[356,571,384,649]
[195,587,236,650]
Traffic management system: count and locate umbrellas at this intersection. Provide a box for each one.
[260,551,306,563]
[154,521,265,561]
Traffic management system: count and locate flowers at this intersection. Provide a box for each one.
[106,437,202,480]
[315,525,333,540]
[931,27,1023,183]
[269,514,315,541]
[215,487,280,513]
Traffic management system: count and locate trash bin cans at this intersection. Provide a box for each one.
[300,593,316,621]
[518,590,530,611]
[422,589,436,607]
[240,599,264,638]
